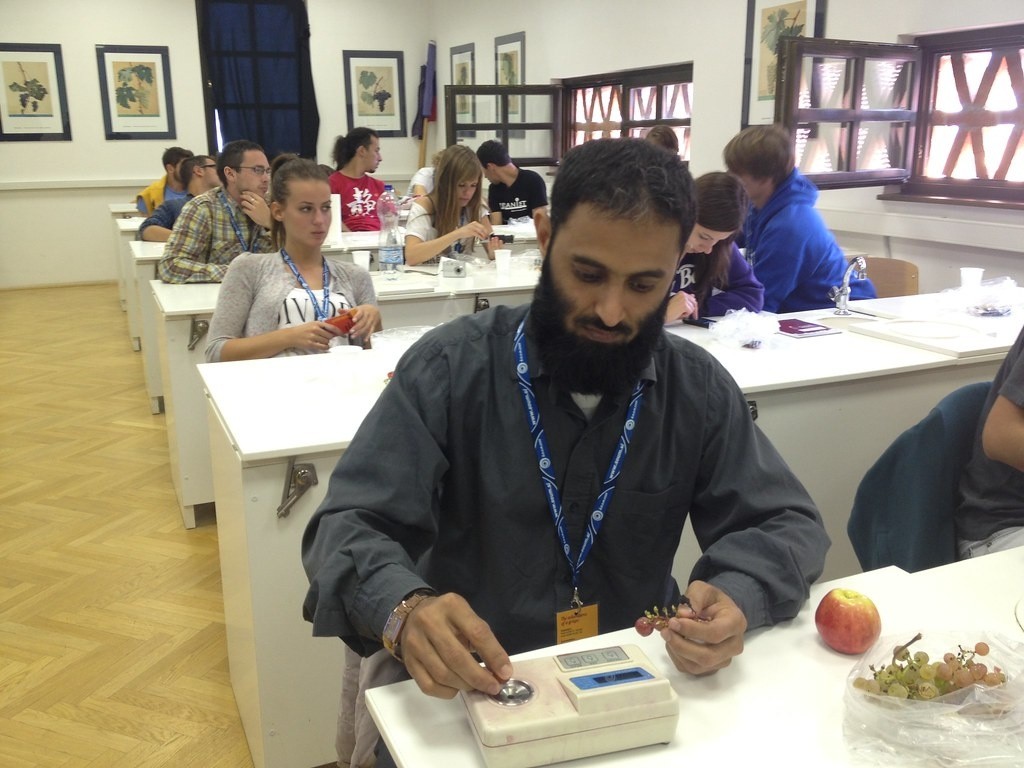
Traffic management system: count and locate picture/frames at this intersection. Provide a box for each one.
[343,50,408,138]
[0,43,72,143]
[741,0,828,140]
[96,44,176,140]
[450,43,476,138]
[495,31,526,140]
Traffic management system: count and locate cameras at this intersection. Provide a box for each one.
[442,260,466,278]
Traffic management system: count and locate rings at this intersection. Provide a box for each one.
[251,198,255,203]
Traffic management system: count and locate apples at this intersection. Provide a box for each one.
[815,589,881,654]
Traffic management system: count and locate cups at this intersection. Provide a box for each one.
[960,267,985,291]
[328,344,364,384]
[493,249,512,274]
[350,251,371,273]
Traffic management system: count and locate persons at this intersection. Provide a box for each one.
[301,136,833,768]
[133,125,876,364]
[953,320,1024,558]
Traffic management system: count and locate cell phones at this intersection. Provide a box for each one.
[682,316,718,329]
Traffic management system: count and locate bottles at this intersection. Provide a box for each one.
[376,184,404,281]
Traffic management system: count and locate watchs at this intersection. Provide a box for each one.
[382,592,436,663]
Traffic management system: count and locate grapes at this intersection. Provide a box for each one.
[853,642,1006,701]
[635,616,666,637]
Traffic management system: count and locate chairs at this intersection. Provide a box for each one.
[334,544,433,767]
[850,258,920,299]
[846,382,995,574]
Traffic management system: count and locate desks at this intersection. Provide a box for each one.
[109,200,1024,766]
